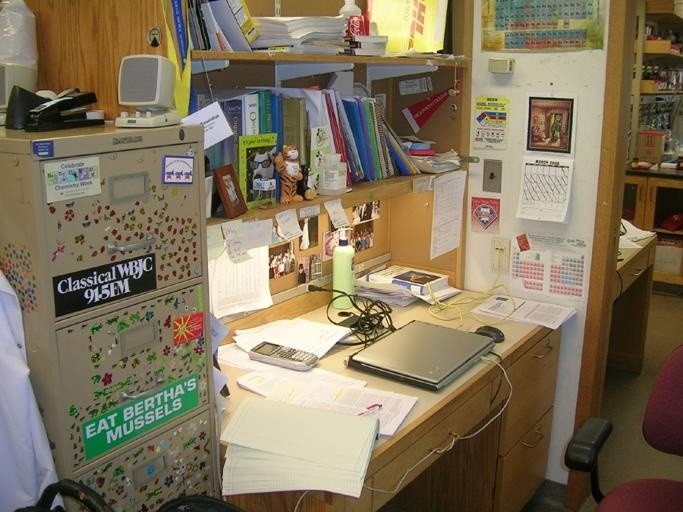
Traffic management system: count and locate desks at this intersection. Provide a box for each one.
[220,289,562,512]
[612,235,659,376]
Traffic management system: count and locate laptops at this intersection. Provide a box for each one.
[343,319,495,393]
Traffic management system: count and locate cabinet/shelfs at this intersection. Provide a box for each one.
[0,120,220,512]
[25,0,475,347]
[621,175,683,297]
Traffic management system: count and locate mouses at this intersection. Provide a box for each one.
[475,325,504,343]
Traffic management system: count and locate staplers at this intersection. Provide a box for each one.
[26,89,105,132]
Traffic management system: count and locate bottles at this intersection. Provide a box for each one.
[318,153,348,196]
[347,17,364,37]
[339,0,362,37]
[333,239,355,310]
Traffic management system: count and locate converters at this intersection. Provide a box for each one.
[338,316,361,328]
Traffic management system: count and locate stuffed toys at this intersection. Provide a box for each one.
[271,147,306,203]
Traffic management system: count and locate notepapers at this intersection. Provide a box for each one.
[207,198,350,266]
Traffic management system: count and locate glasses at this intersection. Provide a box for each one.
[250,159,273,169]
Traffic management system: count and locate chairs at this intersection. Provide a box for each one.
[564,343,683,512]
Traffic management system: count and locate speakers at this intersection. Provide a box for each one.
[114,55,180,127]
[0,61,38,125]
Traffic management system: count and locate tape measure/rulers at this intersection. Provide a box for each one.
[460,156,480,164]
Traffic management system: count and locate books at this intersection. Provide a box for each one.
[190,85,460,217]
[218,398,379,499]
[355,265,460,305]
[187,1,389,56]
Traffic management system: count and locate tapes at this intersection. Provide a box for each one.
[35,89,58,101]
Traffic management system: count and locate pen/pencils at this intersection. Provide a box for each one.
[356,403,383,417]
